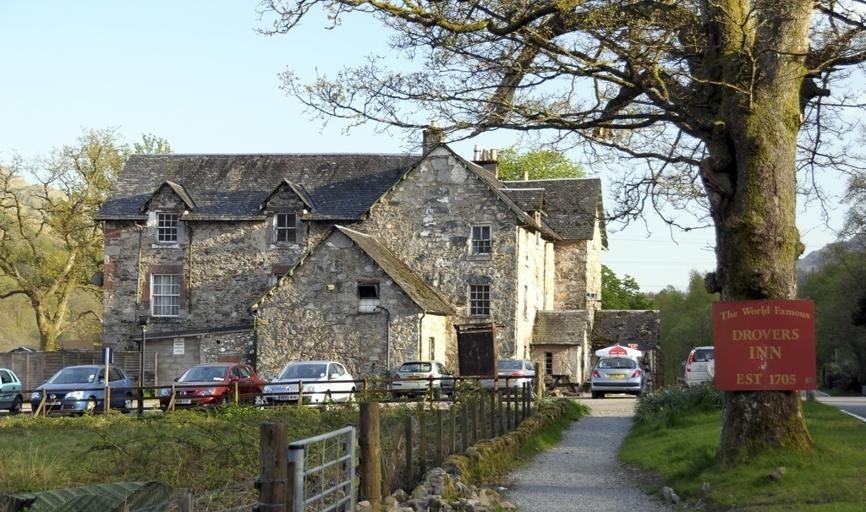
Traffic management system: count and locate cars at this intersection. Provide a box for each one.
[386,361,460,402]
[497,358,536,392]
[590,355,645,397]
[158,362,264,408]
[30,364,134,417]
[0,368,23,414]
[263,360,356,405]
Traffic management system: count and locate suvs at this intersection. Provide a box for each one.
[685,346,714,390]
